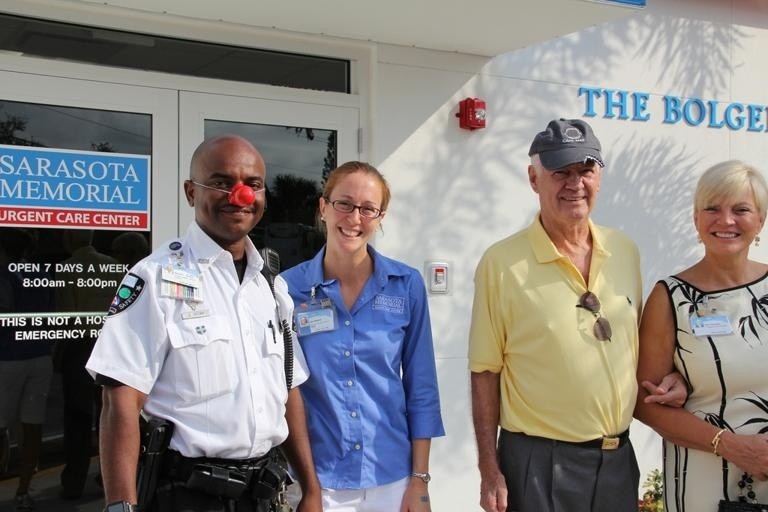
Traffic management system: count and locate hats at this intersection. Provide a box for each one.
[530,118,604,171]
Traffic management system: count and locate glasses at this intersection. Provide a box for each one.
[576,291,612,343]
[325,197,382,219]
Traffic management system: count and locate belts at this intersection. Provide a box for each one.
[557,428,630,450]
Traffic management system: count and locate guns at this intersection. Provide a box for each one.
[138,411,166,512]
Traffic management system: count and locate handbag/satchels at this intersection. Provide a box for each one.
[127,417,174,505]
